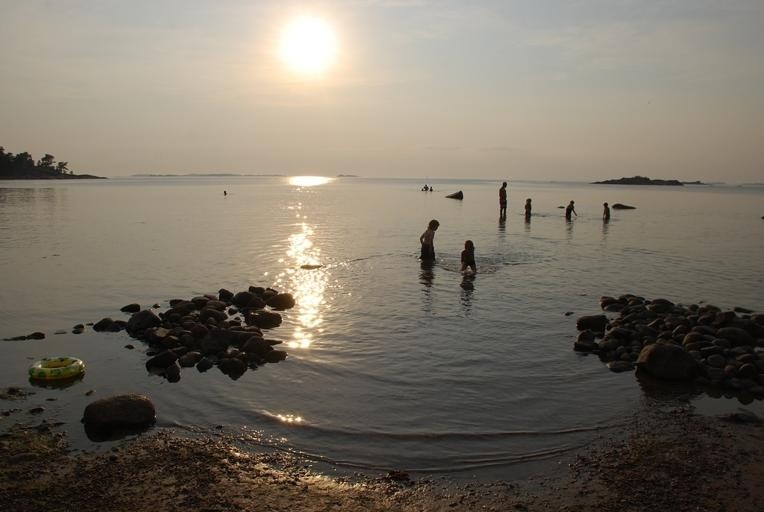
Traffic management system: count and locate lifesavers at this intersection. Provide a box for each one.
[29,357,87,379]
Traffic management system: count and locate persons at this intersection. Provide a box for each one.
[224,190,227,196]
[422,185,433,191]
[603,203,610,225]
[525,198,531,218]
[566,201,577,222]
[419,219,440,261]
[460,240,476,271]
[499,182,507,219]
